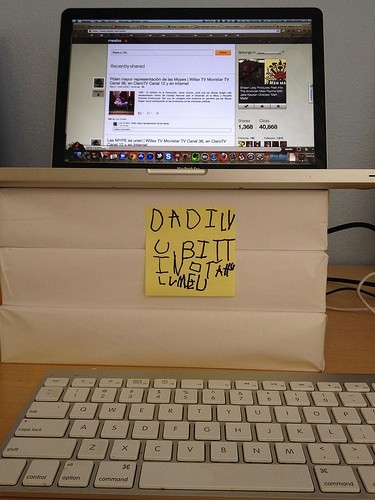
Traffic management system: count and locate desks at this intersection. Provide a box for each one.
[0,259,375,457]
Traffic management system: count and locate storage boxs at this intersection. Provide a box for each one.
[0,245,328,314]
[1,183,329,254]
[0,304,327,373]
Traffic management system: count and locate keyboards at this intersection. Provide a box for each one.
[2,371,375,499]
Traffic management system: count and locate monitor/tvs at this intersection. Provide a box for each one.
[51,8,327,168]
